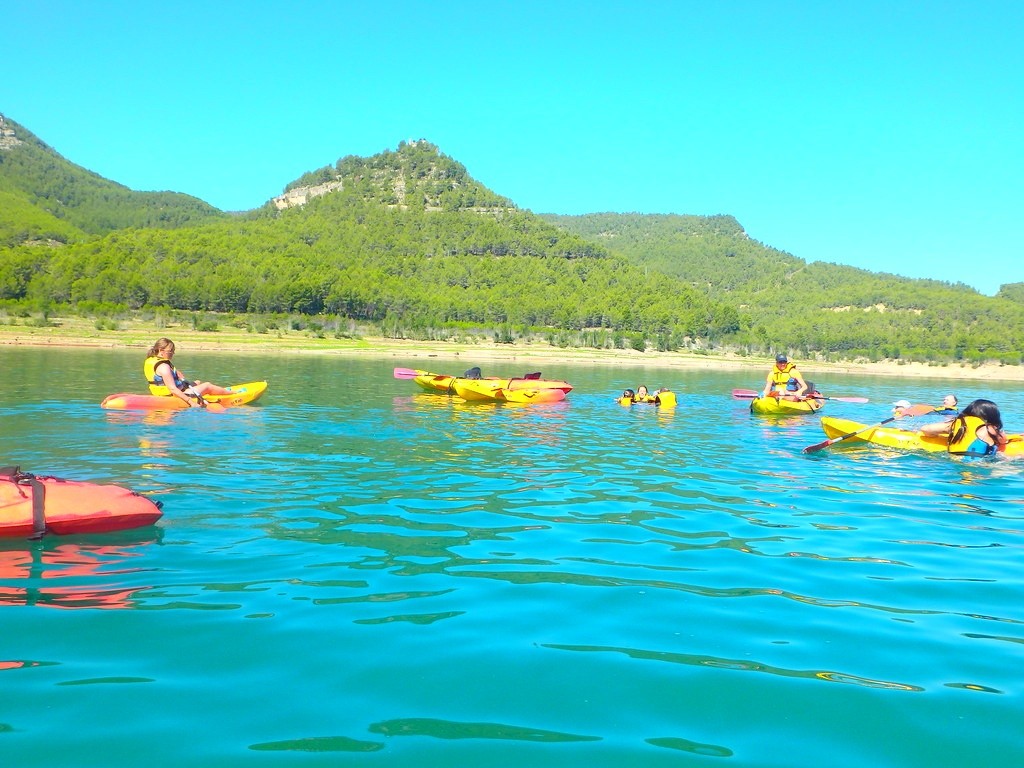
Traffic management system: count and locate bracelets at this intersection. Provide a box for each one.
[186,397,190,402]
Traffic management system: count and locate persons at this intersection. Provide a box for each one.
[618,389,636,404]
[758,354,808,401]
[921,399,1007,463]
[893,400,911,416]
[925,395,958,415]
[464,367,484,380]
[144,338,247,407]
[655,388,677,409]
[633,386,656,402]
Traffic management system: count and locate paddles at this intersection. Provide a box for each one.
[179,381,224,414]
[393,367,454,380]
[730,387,870,404]
[803,404,931,455]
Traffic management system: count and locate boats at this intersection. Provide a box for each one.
[408,372,579,401]
[0,465,166,539]
[101,381,269,413]
[749,390,827,421]
[815,409,1024,460]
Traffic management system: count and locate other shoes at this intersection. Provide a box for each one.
[227,385,231,391]
[237,387,246,393]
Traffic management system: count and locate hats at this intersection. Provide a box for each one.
[893,400,910,409]
[660,387,669,392]
[776,354,789,363]
